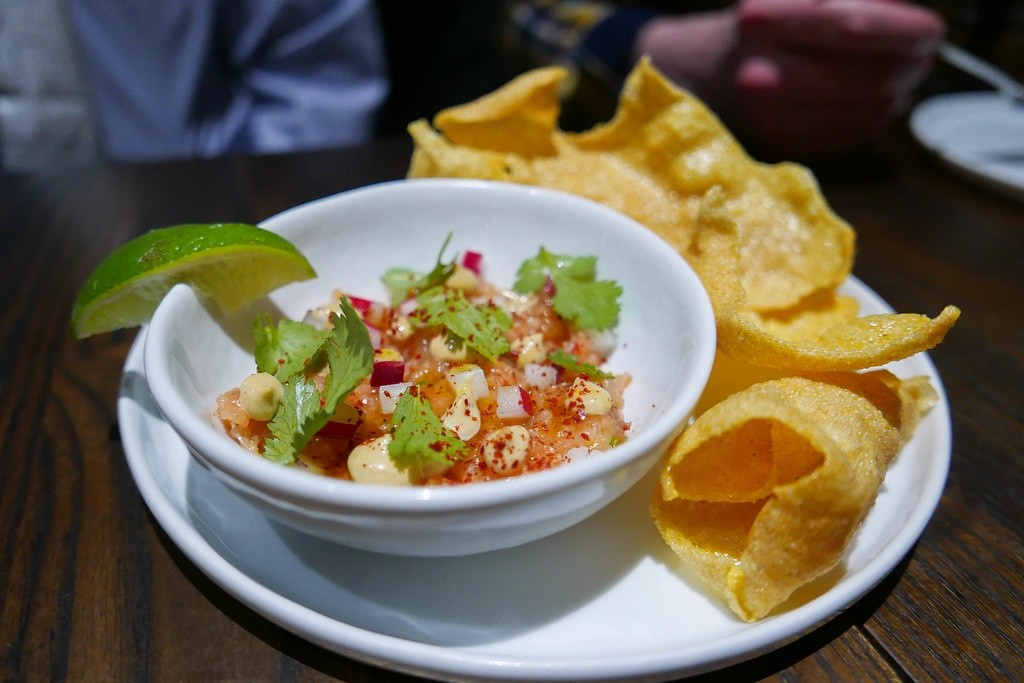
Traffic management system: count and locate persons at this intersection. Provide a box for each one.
[454,1,1023,160]
[0,0,389,171]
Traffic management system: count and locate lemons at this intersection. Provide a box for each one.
[70,221,316,342]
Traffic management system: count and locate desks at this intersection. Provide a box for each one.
[0,154,1024,683]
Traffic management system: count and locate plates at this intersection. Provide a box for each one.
[120,278,954,683]
[910,93,1024,195]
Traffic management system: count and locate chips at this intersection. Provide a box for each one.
[406,58,957,621]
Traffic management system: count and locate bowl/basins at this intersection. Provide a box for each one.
[142,179,717,552]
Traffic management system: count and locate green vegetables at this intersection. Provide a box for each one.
[254,233,622,466]
